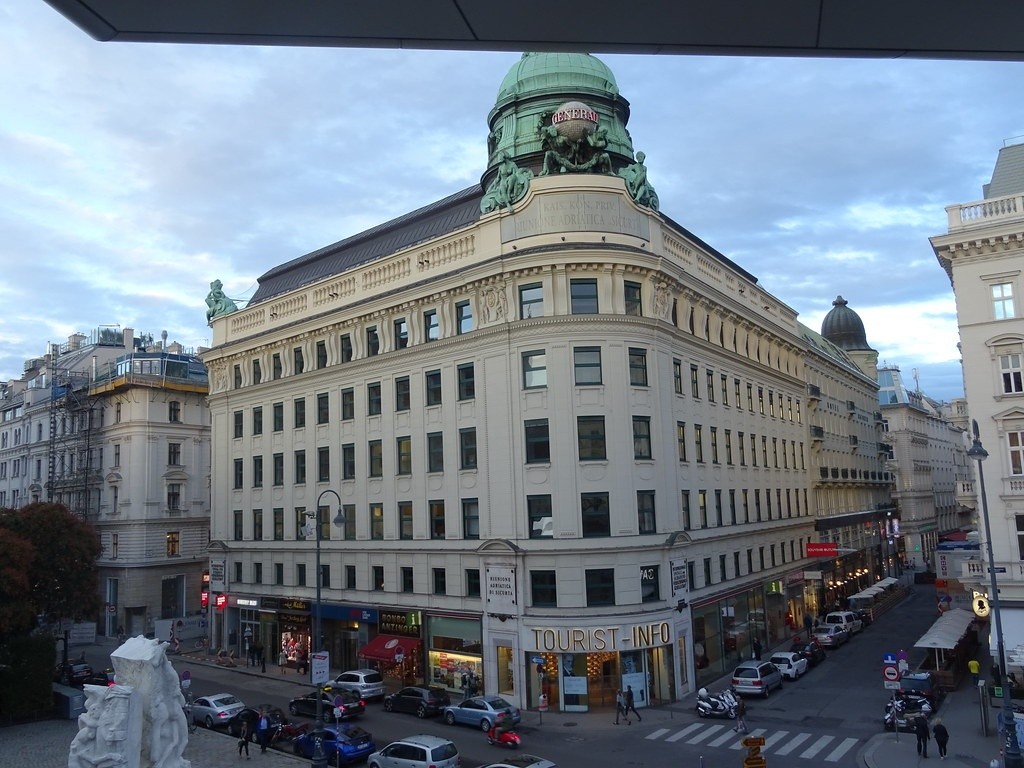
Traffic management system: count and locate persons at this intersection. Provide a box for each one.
[493,708,514,742]
[300,650,308,675]
[732,695,749,734]
[933,717,949,760]
[752,597,840,661]
[278,649,288,675]
[256,707,271,753]
[623,685,641,721]
[239,722,251,760]
[493,126,647,214]
[249,640,256,667]
[255,639,266,666]
[967,655,981,689]
[613,689,631,725]
[116,624,124,646]
[462,670,480,700]
[296,650,303,674]
[914,712,930,758]
[173,620,184,654]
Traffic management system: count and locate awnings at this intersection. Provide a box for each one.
[959,501,978,509]
[358,635,420,662]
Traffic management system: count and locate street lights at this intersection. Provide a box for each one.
[966,419,1024,768]
[302,490,346,767]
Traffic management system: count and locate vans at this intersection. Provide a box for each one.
[826,611,865,636]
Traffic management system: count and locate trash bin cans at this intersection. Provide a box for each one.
[538,694,549,712]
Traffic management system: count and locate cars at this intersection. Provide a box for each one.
[812,625,850,648]
[366,734,460,768]
[444,695,522,732]
[288,687,366,722]
[790,642,824,666]
[479,754,557,768]
[732,660,783,697]
[54,658,285,744]
[292,724,377,765]
[383,685,451,719]
[325,669,385,702]
[768,651,808,680]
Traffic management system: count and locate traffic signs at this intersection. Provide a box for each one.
[530,656,546,664]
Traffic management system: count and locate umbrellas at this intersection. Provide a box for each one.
[847,577,899,608]
[913,608,976,671]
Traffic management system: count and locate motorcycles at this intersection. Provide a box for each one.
[695,686,738,719]
[266,721,309,748]
[885,697,932,731]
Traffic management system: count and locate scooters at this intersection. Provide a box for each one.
[487,722,521,747]
